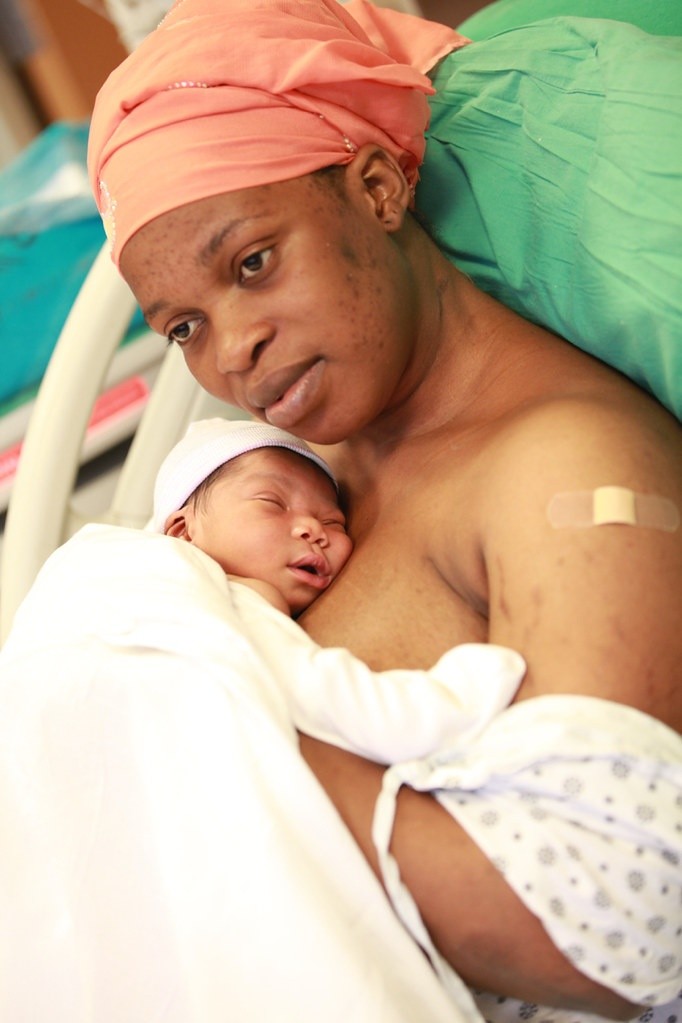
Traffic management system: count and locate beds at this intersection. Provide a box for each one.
[2,0,682,690]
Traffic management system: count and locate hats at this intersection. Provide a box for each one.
[147,418,340,536]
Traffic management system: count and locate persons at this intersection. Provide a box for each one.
[86,2,681,1023]
[140,413,352,619]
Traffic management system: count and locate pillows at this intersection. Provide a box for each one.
[418,17,681,420]
